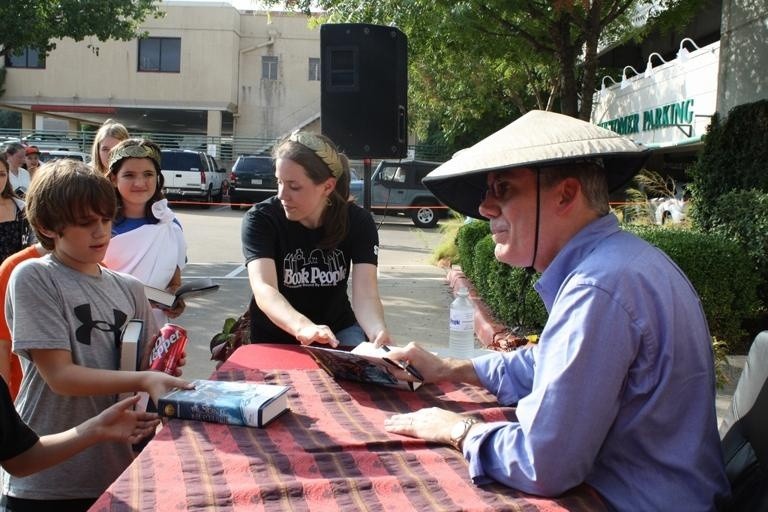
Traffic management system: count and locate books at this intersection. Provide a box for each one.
[132,277,220,311]
[158,379,295,430]
[134,380,157,415]
[300,335,439,390]
[112,320,144,411]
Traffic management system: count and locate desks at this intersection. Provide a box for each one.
[85,344,613,512]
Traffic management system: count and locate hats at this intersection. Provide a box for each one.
[421,109,654,221]
[25,146,41,156]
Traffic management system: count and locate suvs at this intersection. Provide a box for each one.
[228,155,283,211]
[158,150,227,209]
[349,161,453,230]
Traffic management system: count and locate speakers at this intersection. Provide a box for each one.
[321,23,408,159]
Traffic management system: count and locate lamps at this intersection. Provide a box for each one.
[621,65,644,90]
[643,52,672,79]
[107,91,114,107]
[676,38,708,62]
[599,76,620,98]
[33,91,42,104]
[71,92,81,103]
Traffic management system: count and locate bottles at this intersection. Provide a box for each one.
[448,287,477,363]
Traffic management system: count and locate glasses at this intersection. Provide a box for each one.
[479,172,557,200]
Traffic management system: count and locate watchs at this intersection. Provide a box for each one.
[449,416,479,456]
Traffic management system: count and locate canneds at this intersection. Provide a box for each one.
[149,322,188,376]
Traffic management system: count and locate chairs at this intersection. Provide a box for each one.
[718,330,768,512]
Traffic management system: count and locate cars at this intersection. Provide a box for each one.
[0,133,81,151]
[649,197,690,226]
[37,150,95,170]
[0,137,21,147]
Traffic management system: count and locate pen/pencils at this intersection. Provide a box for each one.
[379,342,426,384]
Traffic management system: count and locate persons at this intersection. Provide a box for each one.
[99,136,187,318]
[86,120,129,178]
[239,130,387,349]
[0,140,43,267]
[1,158,196,511]
[378,153,733,510]
[0,367,159,490]
